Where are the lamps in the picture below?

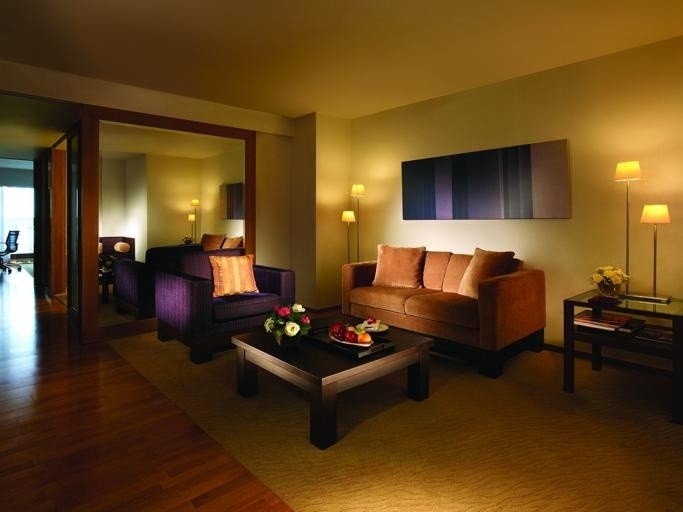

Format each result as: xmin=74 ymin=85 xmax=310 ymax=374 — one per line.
xmin=341 ymin=211 xmax=356 ymax=263
xmin=188 ymin=215 xmax=195 ymax=243
xmin=350 ymin=184 xmax=366 ymax=262
xmin=191 ymin=199 xmax=200 ymax=243
xmin=640 ymin=204 xmax=675 ymax=297
xmin=614 ymin=161 xmax=642 ymax=291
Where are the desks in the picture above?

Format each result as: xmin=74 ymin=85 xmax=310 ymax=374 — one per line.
xmin=98 ymin=276 xmax=112 ymax=305
xmin=563 ymin=288 xmax=683 ymax=426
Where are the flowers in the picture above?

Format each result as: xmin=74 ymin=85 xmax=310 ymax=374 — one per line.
xmin=264 ymin=303 xmax=312 ymax=338
xmin=591 ymin=265 xmax=629 ymax=287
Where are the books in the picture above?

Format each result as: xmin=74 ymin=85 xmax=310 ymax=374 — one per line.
xmin=572 ymin=309 xmax=645 ymax=341
xmin=634 ymin=324 xmax=672 ymax=344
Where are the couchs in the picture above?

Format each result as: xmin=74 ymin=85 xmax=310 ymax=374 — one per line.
xmin=341 ymin=260 xmax=546 ymax=378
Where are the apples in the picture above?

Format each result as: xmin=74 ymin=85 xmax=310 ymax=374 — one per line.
xmin=331 ymin=323 xmax=345 ymax=333
xmin=343 ymin=331 xmax=357 ymax=342
xmin=354 ymin=330 xmax=362 ymax=335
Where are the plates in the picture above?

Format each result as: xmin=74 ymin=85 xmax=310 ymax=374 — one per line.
xmin=356 ymin=323 xmax=389 ymax=332
xmin=329 ymin=328 xmax=374 ymax=347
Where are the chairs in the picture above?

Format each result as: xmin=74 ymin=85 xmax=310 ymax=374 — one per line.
xmin=113 ymin=246 xmax=200 ymax=319
xmin=0 ymin=231 xmax=21 ymax=274
xmin=154 ymin=266 xmax=295 ymax=364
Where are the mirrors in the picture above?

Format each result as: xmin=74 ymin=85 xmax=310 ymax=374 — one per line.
xmin=98 ymin=119 xmax=245 ymax=330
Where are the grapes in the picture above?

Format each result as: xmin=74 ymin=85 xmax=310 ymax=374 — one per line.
xmin=334 ymin=331 xmax=345 ymax=341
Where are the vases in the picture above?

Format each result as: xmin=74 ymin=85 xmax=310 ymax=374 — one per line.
xmin=599 ymin=282 xmax=620 ymax=296
xmin=273 ymin=335 xmax=294 ymax=347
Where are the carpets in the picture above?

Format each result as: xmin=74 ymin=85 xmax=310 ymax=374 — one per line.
xmin=108 ymin=311 xmax=683 ymax=512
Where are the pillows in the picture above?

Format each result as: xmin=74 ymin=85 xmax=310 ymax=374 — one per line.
xmin=201 ymin=234 xmax=226 ymax=251
xmin=222 ymin=237 xmax=243 ymax=248
xmin=367 ymin=244 xmax=515 ymax=301
xmin=208 ymin=254 xmax=260 ymax=298
xmin=177 ymin=249 xmax=246 ymax=303
